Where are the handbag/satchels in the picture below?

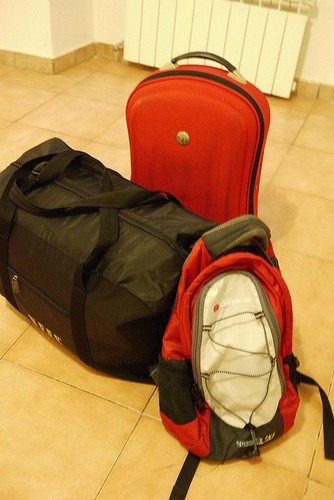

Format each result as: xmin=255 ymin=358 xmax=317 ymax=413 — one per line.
xmin=0 ymin=137 xmax=219 ymax=384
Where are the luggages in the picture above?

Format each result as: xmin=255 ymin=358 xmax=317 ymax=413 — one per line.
xmin=126 ymin=51 xmax=271 ymax=221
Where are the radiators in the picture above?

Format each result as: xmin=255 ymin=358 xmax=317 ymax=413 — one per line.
xmin=123 ymin=0 xmax=308 ymax=99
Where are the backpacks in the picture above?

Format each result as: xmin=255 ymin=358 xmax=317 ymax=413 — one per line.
xmin=158 ymin=215 xmax=300 ymax=461
